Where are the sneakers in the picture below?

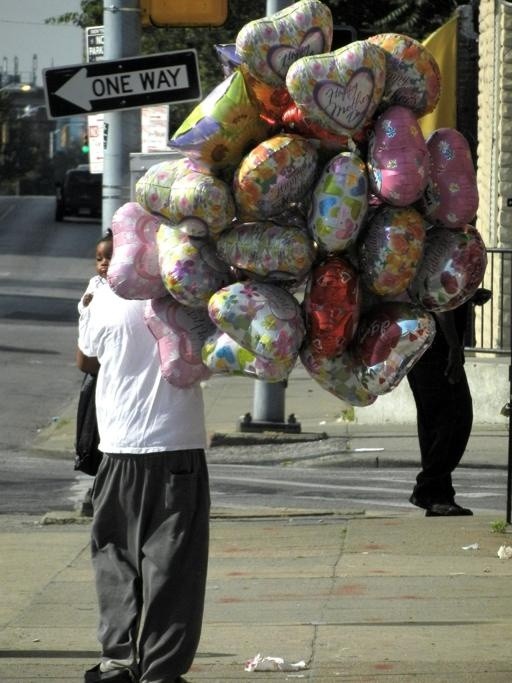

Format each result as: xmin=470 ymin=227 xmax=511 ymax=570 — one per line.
xmin=408 ymin=492 xmax=472 ymax=516
xmin=84 ymin=663 xmax=132 ymax=682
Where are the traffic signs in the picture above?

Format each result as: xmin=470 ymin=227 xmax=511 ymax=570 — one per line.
xmin=39 ymin=50 xmax=201 ymax=120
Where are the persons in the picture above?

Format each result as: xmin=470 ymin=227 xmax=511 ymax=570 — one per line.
xmin=77 ymin=228 xmax=114 ymax=312
xmin=75 ymin=281 xmax=213 ymax=683
xmin=407 ymin=288 xmax=490 ymax=518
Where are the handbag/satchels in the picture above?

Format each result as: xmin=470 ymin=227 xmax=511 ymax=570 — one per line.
xmin=74 ymin=370 xmax=104 ymax=476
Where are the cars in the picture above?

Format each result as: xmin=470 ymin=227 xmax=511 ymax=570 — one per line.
xmin=54 ymin=168 xmax=103 ymax=223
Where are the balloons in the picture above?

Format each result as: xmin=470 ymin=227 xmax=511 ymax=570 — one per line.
xmin=107 ymin=0 xmax=489 ymax=405
